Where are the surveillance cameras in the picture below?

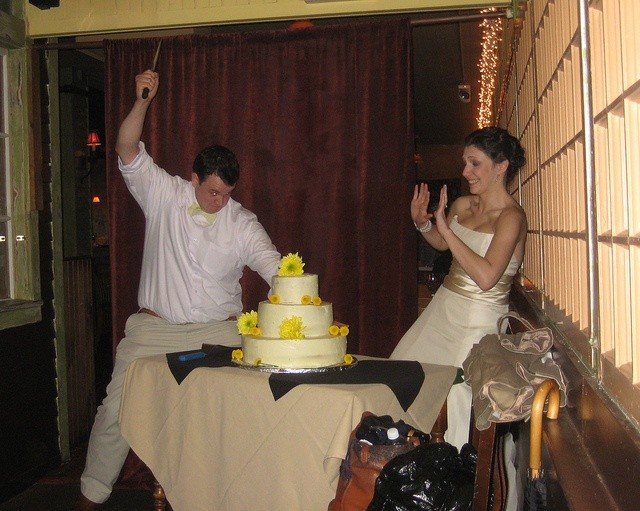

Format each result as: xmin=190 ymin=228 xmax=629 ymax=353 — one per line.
xmin=458 ymin=84 xmax=471 ymax=103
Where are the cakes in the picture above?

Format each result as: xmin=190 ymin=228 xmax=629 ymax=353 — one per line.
xmin=232 ymin=252 xmax=353 ymax=369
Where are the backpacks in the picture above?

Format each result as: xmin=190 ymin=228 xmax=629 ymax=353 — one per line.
xmin=367 ymin=444 xmax=479 ymax=510
xmin=327 ymin=408 xmax=429 ymax=509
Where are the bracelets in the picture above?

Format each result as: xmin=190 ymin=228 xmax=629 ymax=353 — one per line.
xmin=414 ymin=220 xmax=432 ymax=233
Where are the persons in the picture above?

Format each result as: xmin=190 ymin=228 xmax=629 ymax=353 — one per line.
xmin=384 ymin=126 xmax=529 ymax=454
xmin=77 ymin=69 xmax=283 ymax=511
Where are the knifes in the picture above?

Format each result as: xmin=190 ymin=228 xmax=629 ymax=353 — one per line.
xmin=141 ymin=41 xmax=163 ymax=100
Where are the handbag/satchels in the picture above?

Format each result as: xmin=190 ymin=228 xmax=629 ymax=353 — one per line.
xmin=461 ymin=312 xmax=568 ymax=431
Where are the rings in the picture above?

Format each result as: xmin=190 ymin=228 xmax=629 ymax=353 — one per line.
xmin=445 ymin=204 xmax=448 ymax=208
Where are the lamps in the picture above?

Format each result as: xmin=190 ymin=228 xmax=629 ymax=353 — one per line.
xmin=87 ymin=131 xmax=102 ymax=151
